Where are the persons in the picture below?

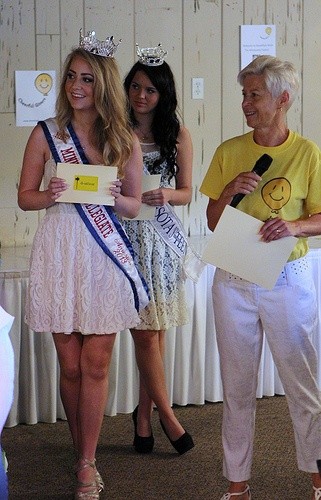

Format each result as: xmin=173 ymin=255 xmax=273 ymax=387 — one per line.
xmin=199 ymin=55 xmax=321 ymax=500
xmin=18 ymin=47 xmax=152 ymax=500
xmin=104 ymin=55 xmax=195 ymax=455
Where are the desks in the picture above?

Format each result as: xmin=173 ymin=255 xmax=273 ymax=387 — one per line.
xmin=0 ymin=234 xmax=321 ymax=428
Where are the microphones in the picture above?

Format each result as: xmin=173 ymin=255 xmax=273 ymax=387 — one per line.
xmin=230 ymin=153 xmax=273 ymax=208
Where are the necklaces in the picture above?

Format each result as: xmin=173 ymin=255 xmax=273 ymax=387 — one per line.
xmin=135 ymin=125 xmax=151 ymax=140
xmin=75 ymin=129 xmax=91 ymax=149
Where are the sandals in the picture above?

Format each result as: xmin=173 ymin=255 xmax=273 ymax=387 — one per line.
xmin=218 ymin=484 xmax=252 ymax=500
xmin=312 ymin=487 xmax=321 ymax=500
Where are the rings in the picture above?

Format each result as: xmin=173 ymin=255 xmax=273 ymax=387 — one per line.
xmin=276 ymin=228 xmax=280 ymax=233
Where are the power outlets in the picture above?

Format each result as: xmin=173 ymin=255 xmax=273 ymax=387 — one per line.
xmin=190 ymin=77 xmax=205 ymax=99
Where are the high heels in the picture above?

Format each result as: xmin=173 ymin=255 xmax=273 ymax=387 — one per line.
xmin=159 ymin=419 xmax=195 ymax=456
xmin=76 ymin=459 xmax=99 ymax=500
xmin=95 ymin=470 xmax=104 ymax=494
xmin=132 ymin=406 xmax=154 ymax=457
